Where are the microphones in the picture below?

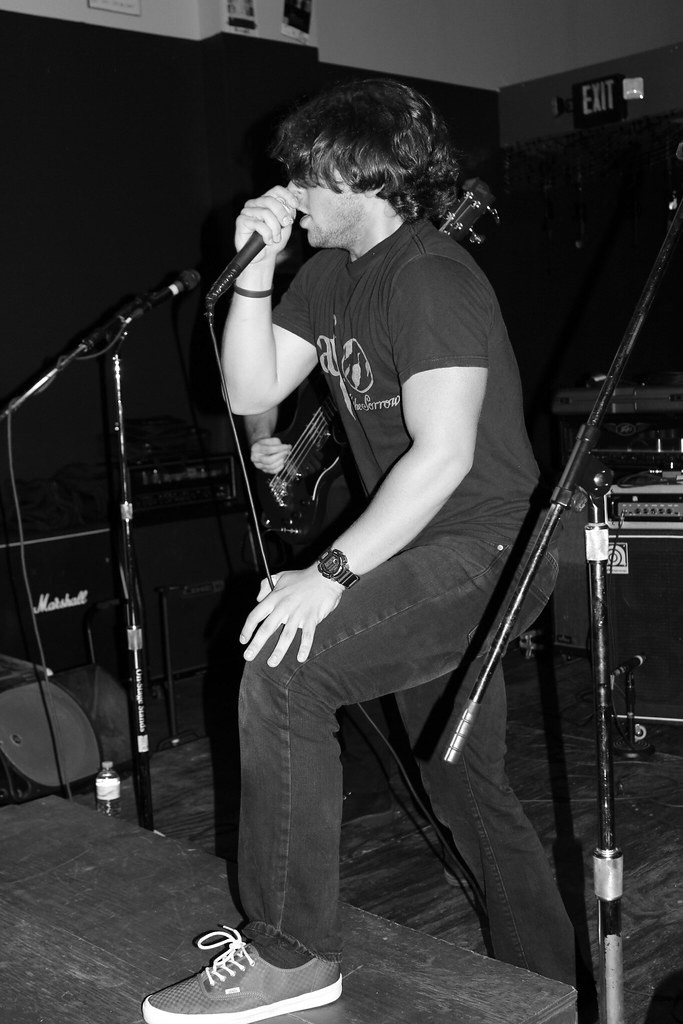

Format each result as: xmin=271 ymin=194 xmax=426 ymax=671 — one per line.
xmin=610 ymin=653 xmax=657 ymax=762
xmin=127 ymin=265 xmax=202 ymax=323
xmin=205 ymin=204 xmax=296 ymax=310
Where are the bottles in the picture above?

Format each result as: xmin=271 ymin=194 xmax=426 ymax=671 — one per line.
xmin=96 ymin=761 xmax=122 ymax=820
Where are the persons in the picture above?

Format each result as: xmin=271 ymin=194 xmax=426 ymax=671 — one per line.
xmin=142 ymin=82 xmax=603 ymax=1024
xmin=236 ymin=209 xmax=484 ymax=890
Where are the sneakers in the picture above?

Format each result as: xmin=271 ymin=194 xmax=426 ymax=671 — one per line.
xmin=140 ymin=926 xmax=343 ymax=1024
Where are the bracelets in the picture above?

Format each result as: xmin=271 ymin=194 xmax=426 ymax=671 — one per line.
xmin=234 ymin=285 xmax=273 ymax=299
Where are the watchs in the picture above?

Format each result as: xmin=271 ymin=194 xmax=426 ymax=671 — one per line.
xmin=320 ymin=548 xmax=360 ymax=590
